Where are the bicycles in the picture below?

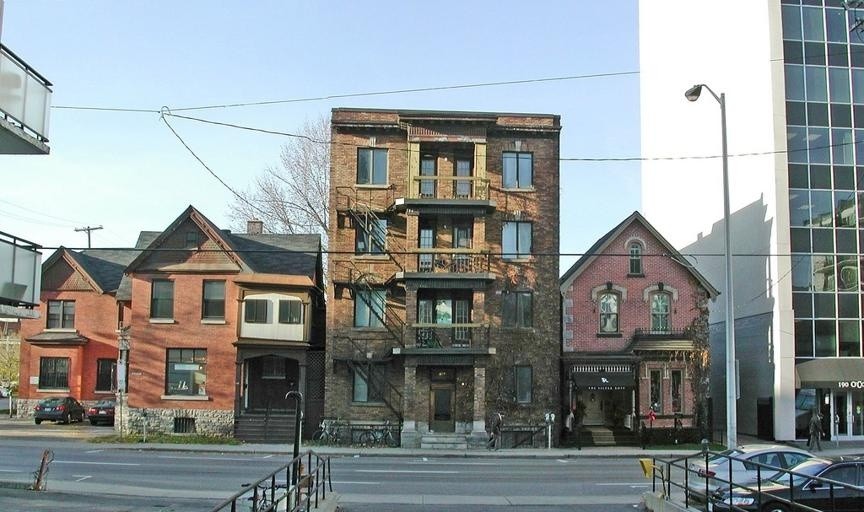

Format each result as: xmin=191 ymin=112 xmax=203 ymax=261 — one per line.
xmin=313 ymin=416 xmax=401 ymax=448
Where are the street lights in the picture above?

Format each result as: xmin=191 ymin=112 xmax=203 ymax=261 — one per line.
xmin=685 ymin=84 xmax=737 ymax=450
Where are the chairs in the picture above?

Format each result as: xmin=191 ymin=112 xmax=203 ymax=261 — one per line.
xmin=770 ymin=456 xmax=781 ymax=467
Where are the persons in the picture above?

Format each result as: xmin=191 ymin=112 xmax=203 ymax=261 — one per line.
xmin=809 ymin=413 xmax=826 ymax=453
xmin=485 ymin=411 xmax=505 ymax=452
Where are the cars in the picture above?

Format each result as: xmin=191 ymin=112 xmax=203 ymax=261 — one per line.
xmin=33 ymin=396 xmax=115 ymax=426
xmin=683 ymin=443 xmax=864 ymax=512
